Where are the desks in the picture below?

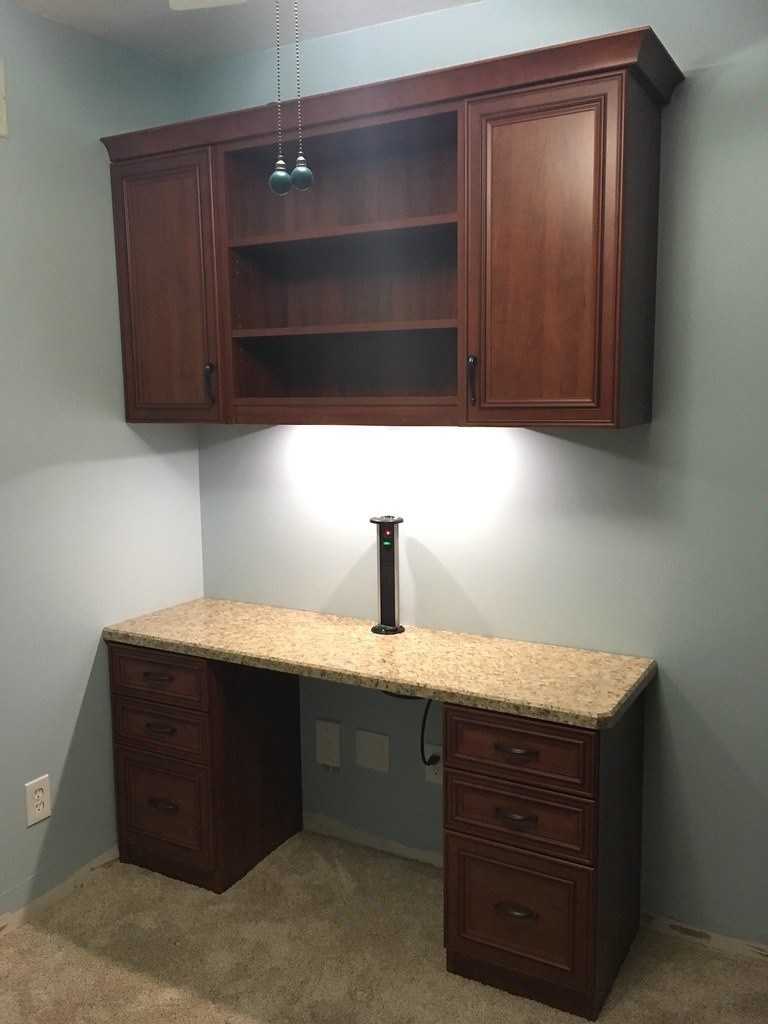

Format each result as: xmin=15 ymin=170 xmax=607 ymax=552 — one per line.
xmin=102 ymin=595 xmax=658 ymax=730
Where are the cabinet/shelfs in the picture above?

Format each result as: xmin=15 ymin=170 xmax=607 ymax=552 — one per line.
xmin=106 ymin=641 xmax=304 ymax=896
xmin=463 ymin=66 xmax=661 ymax=430
xmin=443 ymin=690 xmax=647 ymax=1022
xmin=227 ymin=211 xmax=464 ymax=407
xmin=110 ymin=145 xmax=227 ymax=425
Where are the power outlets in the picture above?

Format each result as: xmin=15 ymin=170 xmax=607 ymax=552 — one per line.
xmin=424 ymin=744 xmax=442 ymax=784
xmin=24 ymin=774 xmax=51 ymax=828
